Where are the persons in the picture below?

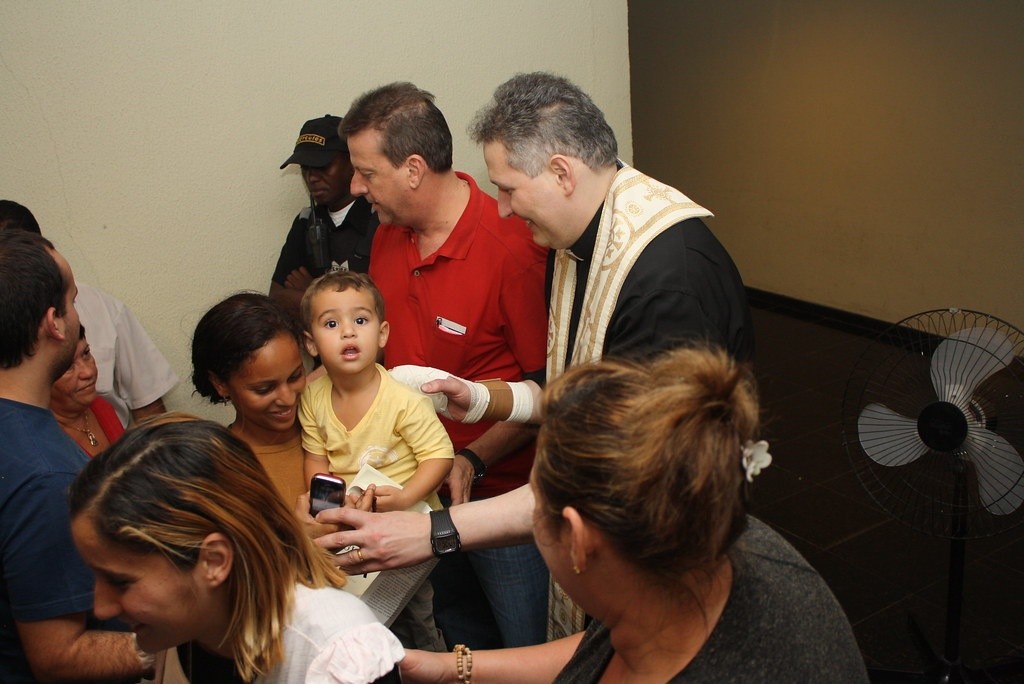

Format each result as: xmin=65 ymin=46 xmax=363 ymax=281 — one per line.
xmin=298 ymin=268 xmax=455 ymax=653
xmin=189 ymin=292 xmax=374 ymax=555
xmin=393 ymin=346 xmax=869 ymax=684
xmin=0 ymin=228 xmax=157 ymax=683
xmin=50 ymin=323 xmax=125 ymax=460
xmin=0 ymin=199 xmax=177 ymax=428
xmin=267 ymin=114 xmax=379 ymax=316
xmin=62 ymin=413 xmax=405 ymax=684
xmin=337 ymin=79 xmax=551 ymax=652
xmin=312 ymin=72 xmax=751 ymax=644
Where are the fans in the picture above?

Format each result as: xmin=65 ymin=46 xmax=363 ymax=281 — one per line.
xmin=837 ymin=306 xmax=1024 ymax=684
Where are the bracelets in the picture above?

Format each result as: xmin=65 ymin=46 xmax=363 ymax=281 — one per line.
xmin=452 ymin=644 xmax=473 ymax=684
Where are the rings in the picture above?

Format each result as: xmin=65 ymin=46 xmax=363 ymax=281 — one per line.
xmin=356 ymin=550 xmax=366 ymax=562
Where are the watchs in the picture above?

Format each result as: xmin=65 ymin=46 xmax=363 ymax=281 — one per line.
xmin=428 ymin=509 xmax=461 ymax=558
xmin=456 ymin=447 xmax=488 ymax=482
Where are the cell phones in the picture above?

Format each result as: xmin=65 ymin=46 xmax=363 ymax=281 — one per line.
xmin=308 ymin=473 xmax=346 ymax=518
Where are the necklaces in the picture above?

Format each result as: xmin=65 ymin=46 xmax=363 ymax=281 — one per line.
xmin=60 ymin=411 xmax=99 ymax=446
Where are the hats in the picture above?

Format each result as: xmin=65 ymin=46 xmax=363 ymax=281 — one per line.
xmin=279 ymin=114 xmax=347 ymax=169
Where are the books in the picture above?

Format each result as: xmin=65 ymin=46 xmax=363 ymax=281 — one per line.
xmin=335 ymin=462 xmax=440 ymax=630
